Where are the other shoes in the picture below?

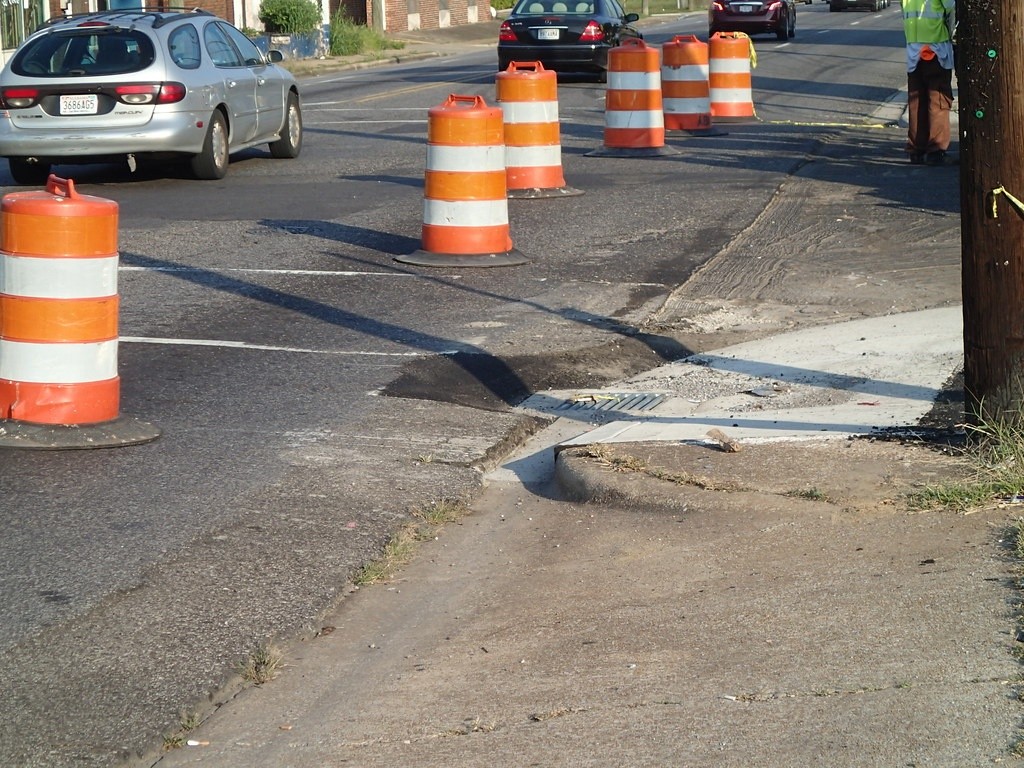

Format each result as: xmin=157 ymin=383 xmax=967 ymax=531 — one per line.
xmin=910 ymin=152 xmax=924 ymax=164
xmin=928 ymin=149 xmax=944 ymax=165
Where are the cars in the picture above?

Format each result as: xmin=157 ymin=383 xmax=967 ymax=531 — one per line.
xmin=827 ymin=0 xmax=891 ymax=12
xmin=708 ymin=0 xmax=797 ymax=39
xmin=498 ymin=0 xmax=643 ymax=82
xmin=0 ymin=7 xmax=304 ymax=181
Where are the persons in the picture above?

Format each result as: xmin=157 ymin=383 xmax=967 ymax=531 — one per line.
xmin=900 ymin=0 xmax=956 ymax=166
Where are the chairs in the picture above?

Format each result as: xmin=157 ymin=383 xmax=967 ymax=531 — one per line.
xmin=93 ymin=40 xmax=131 ymax=75
